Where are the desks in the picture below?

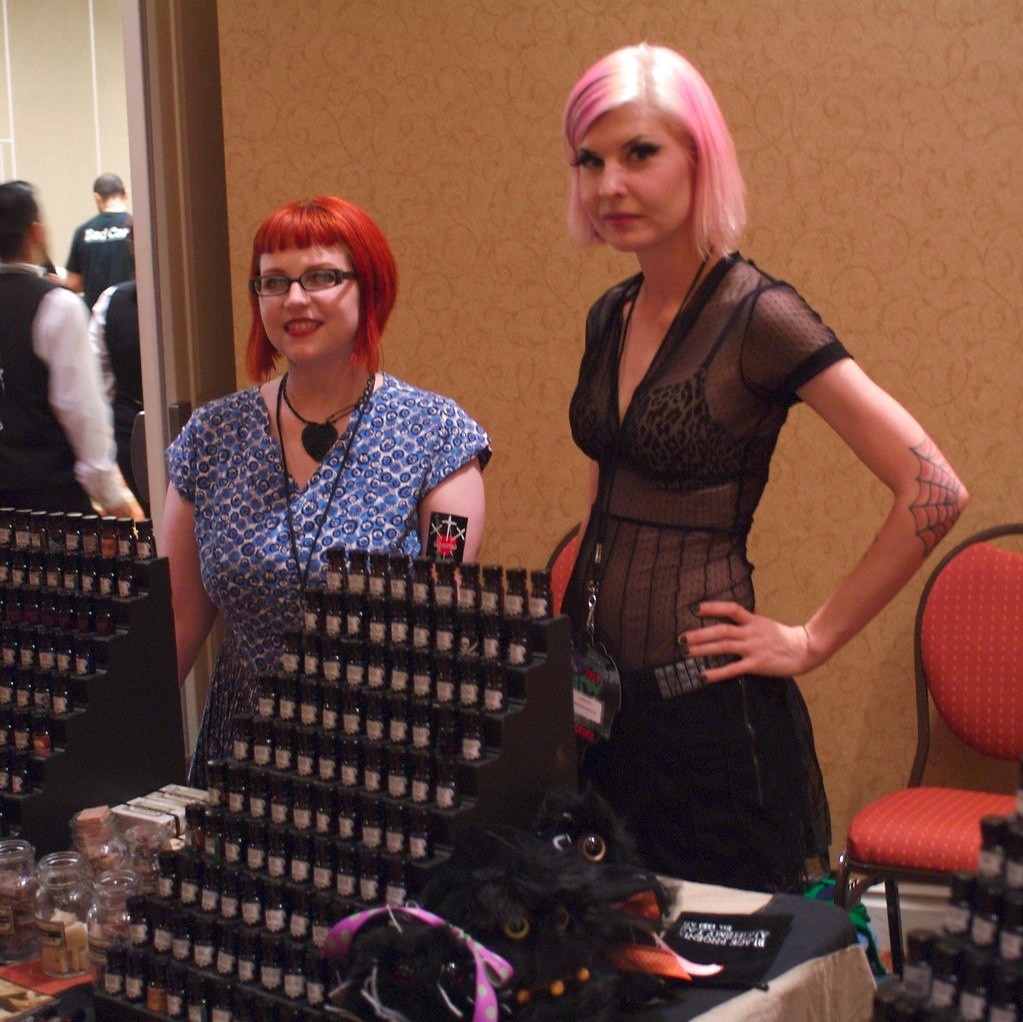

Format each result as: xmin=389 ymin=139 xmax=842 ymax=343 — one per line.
xmin=0 ymin=875 xmax=878 ymax=1022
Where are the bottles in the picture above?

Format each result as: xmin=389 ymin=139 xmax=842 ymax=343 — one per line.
xmin=0 ymin=814 xmax=169 ymax=980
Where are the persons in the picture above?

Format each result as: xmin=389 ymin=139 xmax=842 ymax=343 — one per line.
xmin=560 ymin=43 xmax=972 ymax=898
xmin=89 ymin=218 xmax=152 ymax=518
xmin=156 ymin=197 xmax=494 ymax=795
xmin=47 ymin=173 xmax=134 ymax=312
xmin=0 ymin=181 xmax=145 ymax=527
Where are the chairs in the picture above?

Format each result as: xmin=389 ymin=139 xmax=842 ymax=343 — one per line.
xmin=834 ymin=525 xmax=1023 ymax=980
xmin=546 ymin=523 xmax=583 ymax=618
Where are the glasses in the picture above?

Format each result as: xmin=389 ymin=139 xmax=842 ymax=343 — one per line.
xmin=249 ymin=266 xmax=359 ymax=297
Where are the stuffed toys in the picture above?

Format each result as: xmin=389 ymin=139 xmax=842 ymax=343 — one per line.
xmin=326 ymin=782 xmax=678 ymax=1022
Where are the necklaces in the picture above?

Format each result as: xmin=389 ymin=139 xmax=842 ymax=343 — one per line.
xmin=276 ymin=366 xmax=376 ymax=594
